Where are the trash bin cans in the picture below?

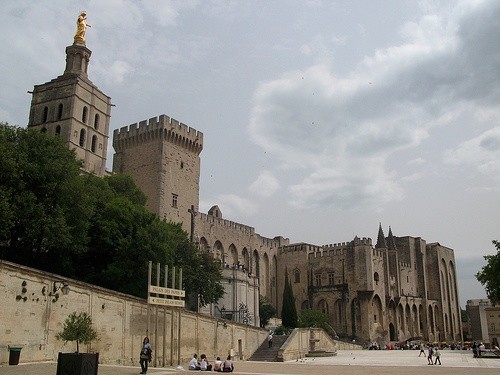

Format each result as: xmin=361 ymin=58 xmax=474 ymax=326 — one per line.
xmin=9 ymin=347 xmax=23 ymax=365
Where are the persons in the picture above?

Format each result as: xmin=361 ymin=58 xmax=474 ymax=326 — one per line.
xmin=418 ymin=342 xmax=426 ymax=357
xmin=200 ymin=354 xmax=212 ymax=371
xmin=268 ymin=333 xmax=273 ymax=348
xmin=189 ymin=353 xmax=201 ymax=371
xmin=248 ymin=272 xmax=253 ymax=279
xmin=362 ymin=343 xmax=398 ymax=350
xmin=283 ymin=332 xmax=285 ymax=335
xmin=139 ymin=337 xmax=151 ymax=375
xmin=223 ymin=261 xmax=244 ymax=270
xmin=399 ymin=342 xmax=450 ymax=350
xmin=428 ymin=345 xmax=434 ymax=365
xmin=450 ymin=342 xmax=485 ymax=356
xmin=223 ymin=355 xmax=234 ymax=372
xmin=75 ymin=10 xmax=91 ymax=41
xmin=493 ymin=344 xmax=500 ymax=351
xmin=214 ymin=357 xmax=222 ymax=371
xmin=434 ymin=347 xmax=441 ymax=365
xmin=243 ymin=267 xmax=247 ymax=271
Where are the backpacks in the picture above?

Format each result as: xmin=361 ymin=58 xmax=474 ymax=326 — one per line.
xmin=214 ymin=364 xmax=219 ymax=370
xmin=201 ymin=362 xmax=207 ymax=370
